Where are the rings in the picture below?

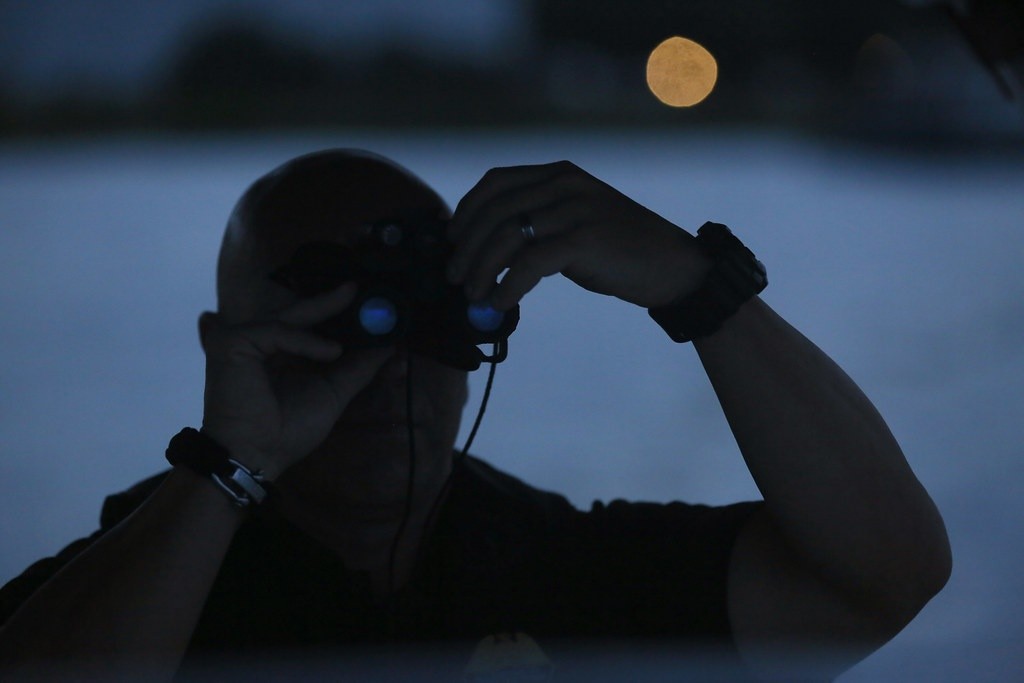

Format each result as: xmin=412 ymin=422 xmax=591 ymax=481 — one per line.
xmin=517 ymin=211 xmax=538 ymax=245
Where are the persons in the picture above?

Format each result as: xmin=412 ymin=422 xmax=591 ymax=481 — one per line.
xmin=1 ymin=149 xmax=949 ymax=681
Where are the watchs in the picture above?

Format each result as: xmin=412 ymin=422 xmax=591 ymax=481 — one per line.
xmin=648 ymin=221 xmax=768 ymax=343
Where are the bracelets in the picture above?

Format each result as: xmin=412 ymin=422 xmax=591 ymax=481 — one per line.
xmin=165 ymin=425 xmax=282 ymax=512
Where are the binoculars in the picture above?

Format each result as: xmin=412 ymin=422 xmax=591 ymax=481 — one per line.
xmin=281 ymin=206 xmax=521 ymax=366
